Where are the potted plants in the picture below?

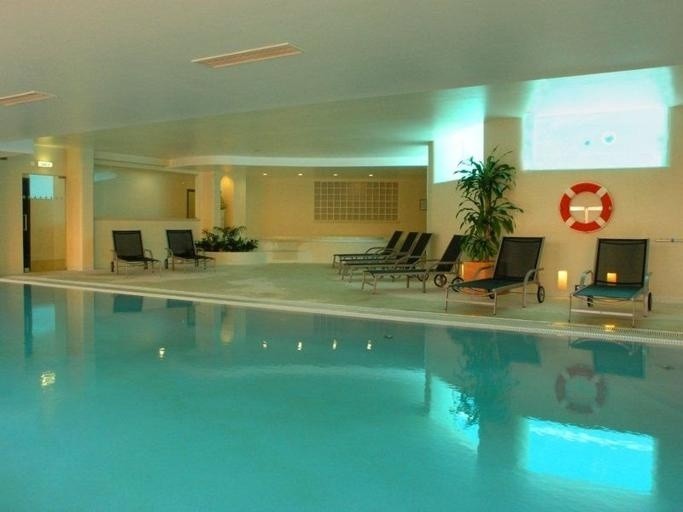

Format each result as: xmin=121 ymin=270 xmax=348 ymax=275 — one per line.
xmin=455 ymin=145 xmax=524 ymax=295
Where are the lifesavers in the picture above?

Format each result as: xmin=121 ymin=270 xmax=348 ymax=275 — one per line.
xmin=560 ymin=183 xmax=611 ymax=231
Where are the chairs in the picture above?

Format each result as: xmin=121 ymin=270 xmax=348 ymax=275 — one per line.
xmin=332 ymin=229 xmax=467 ymax=296
xmin=446 ymin=328 xmax=544 ymax=366
xmin=444 ymin=238 xmax=545 ymax=315
xmin=569 ymin=337 xmax=651 ymax=380
xmin=112 ymin=230 xmax=161 ymax=275
xmin=569 ymin=237 xmax=651 ymax=328
xmin=165 ymin=229 xmax=215 ymax=270
